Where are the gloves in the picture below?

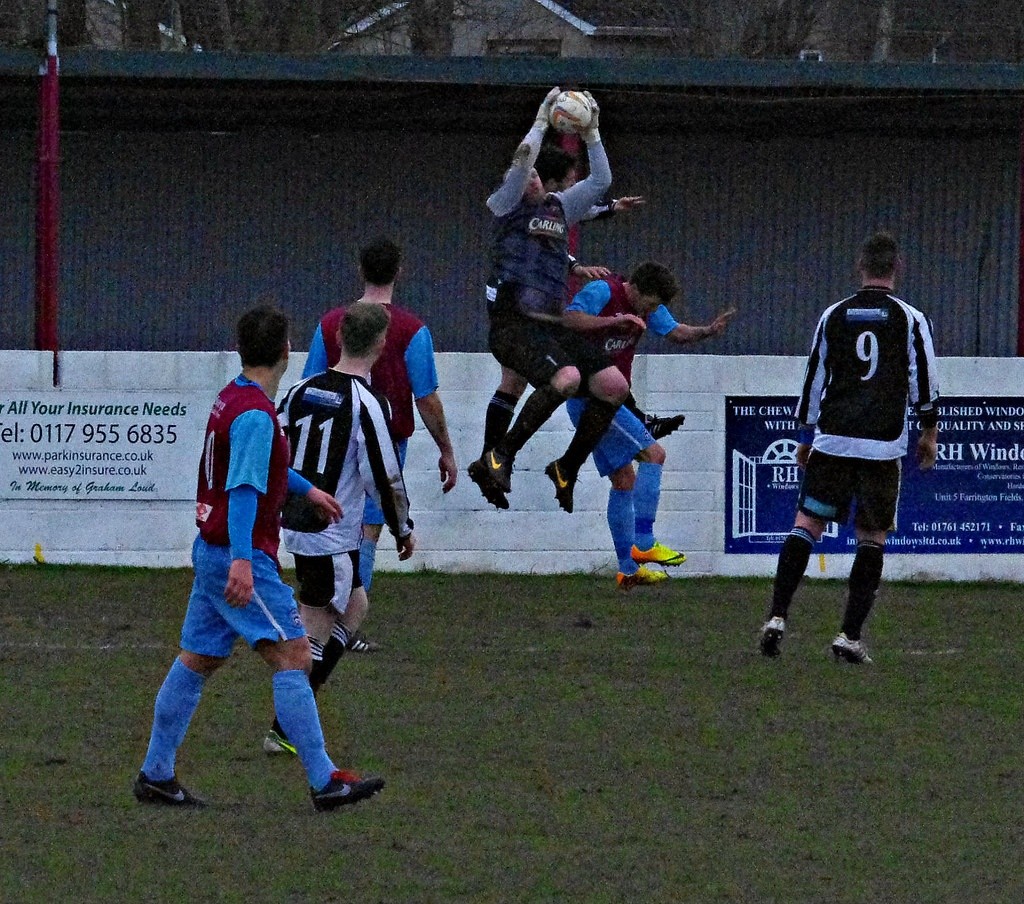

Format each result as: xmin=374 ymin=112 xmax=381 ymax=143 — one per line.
xmin=582 ymin=90 xmax=601 ymax=129
xmin=536 ymin=86 xmax=560 ymax=123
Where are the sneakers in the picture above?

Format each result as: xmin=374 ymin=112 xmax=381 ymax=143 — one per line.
xmin=484 ymin=447 xmax=512 ymax=492
xmin=263 ymin=729 xmax=298 ymax=755
xmin=347 ymin=635 xmax=379 ymax=652
xmin=467 ymin=460 xmax=509 ymax=509
xmin=630 ymin=539 xmax=686 ymax=567
xmin=134 ymin=771 xmax=209 ymax=808
xmin=310 ymin=770 xmax=386 ymax=812
xmin=831 ymin=633 xmax=873 ymax=664
xmin=545 ymin=460 xmax=583 ymax=513
xmin=643 ymin=414 xmax=685 ymax=440
xmin=760 ymin=616 xmax=786 ymax=658
xmin=616 ymin=563 xmax=670 ymax=591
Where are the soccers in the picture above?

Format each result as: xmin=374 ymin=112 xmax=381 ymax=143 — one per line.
xmin=550 ymin=91 xmax=592 ymax=133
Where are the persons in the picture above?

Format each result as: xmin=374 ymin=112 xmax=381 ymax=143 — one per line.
xmin=468 ymin=138 xmax=683 ymax=508
xmin=562 ymin=262 xmax=738 ymax=587
xmin=486 ymin=87 xmax=630 ymax=512
xmin=131 ymin=306 xmax=385 ymax=815
xmin=763 ymin=234 xmax=938 ymax=664
xmin=262 ymin=303 xmax=417 ymax=755
xmin=302 ymin=237 xmax=458 ymax=653
